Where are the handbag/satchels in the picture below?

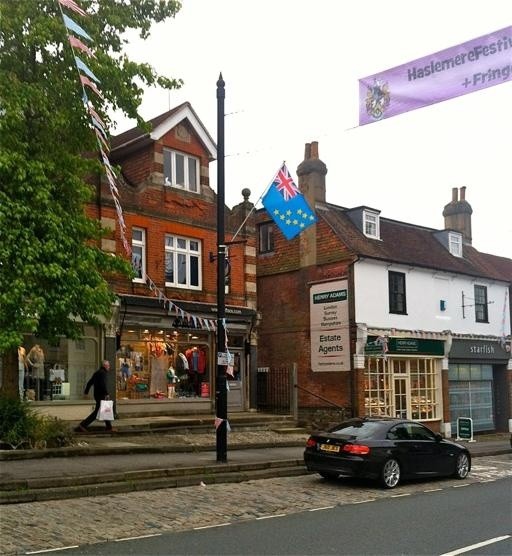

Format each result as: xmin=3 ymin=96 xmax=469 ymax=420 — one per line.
xmin=97 ymin=399 xmax=114 ymax=421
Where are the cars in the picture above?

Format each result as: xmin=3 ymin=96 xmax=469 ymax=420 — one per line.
xmin=303 ymin=416 xmax=472 ymax=490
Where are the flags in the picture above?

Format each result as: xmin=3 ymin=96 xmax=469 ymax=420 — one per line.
xmin=262 ymin=165 xmax=318 ymax=241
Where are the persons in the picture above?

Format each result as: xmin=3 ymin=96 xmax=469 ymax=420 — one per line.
xmin=176 ymin=353 xmax=190 ymax=380
xmin=18 ymin=344 xmax=29 ymax=402
xmin=185 ymin=346 xmax=206 ymax=374
xmin=165 ymin=365 xmax=181 ymax=400
xmin=27 ymin=344 xmax=46 ymax=381
xmin=78 ymin=359 xmax=118 ymax=434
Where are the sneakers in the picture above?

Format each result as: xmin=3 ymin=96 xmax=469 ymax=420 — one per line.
xmin=105 ymin=428 xmax=118 ymax=431
xmin=78 ymin=423 xmax=88 ymax=432
